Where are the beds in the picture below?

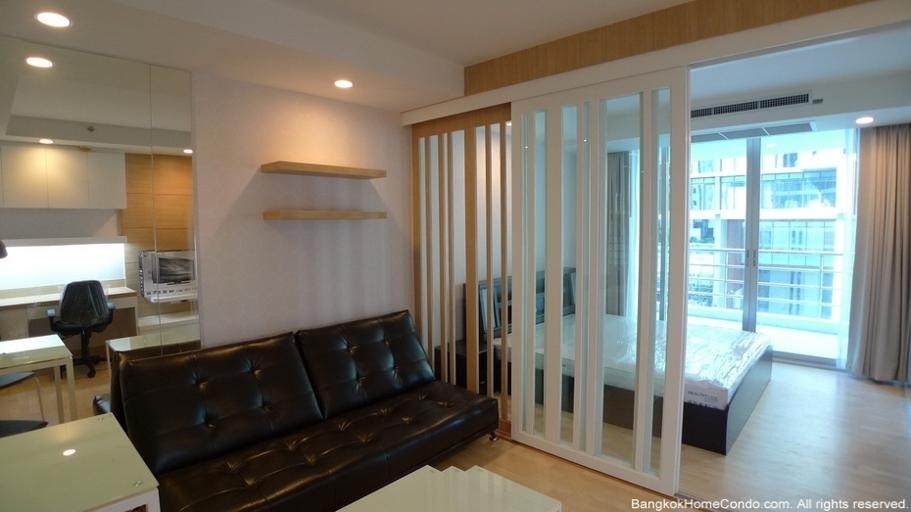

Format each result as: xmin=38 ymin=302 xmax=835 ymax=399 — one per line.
xmin=463 ymin=266 xmax=775 ymax=455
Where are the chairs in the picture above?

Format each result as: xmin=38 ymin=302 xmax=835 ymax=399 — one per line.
xmin=47 ymin=280 xmax=116 ymax=378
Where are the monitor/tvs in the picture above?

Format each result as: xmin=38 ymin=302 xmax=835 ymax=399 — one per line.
xmin=152 ymin=257 xmax=196 ymax=286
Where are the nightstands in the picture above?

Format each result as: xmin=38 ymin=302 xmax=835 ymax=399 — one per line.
xmin=434 ymin=339 xmax=498 ymax=396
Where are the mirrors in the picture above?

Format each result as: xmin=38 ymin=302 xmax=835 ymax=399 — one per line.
xmin=1 ymin=30 xmax=204 ymax=437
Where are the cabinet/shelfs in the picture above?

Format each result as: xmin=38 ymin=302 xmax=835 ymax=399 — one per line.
xmin=261 ymin=161 xmax=389 ymax=222
xmin=0 ymin=142 xmax=127 ymax=209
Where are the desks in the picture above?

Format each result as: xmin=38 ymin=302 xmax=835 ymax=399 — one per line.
xmin=3 ymin=412 xmax=163 ymax=512
xmin=0 ymin=324 xmax=201 ymax=424
xmin=1 ymin=286 xmax=140 ymax=345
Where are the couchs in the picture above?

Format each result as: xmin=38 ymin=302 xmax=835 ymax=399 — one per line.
xmin=121 ymin=307 xmax=501 ymax=512
